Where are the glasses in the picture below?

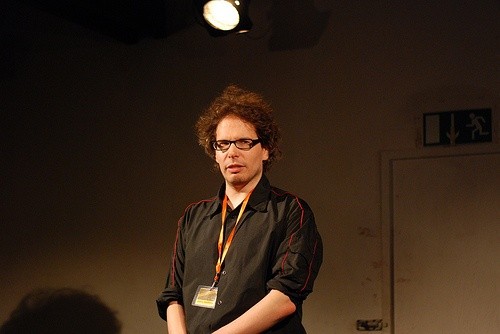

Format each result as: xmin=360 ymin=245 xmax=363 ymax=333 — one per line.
xmin=211 ymin=138 xmax=260 ymax=151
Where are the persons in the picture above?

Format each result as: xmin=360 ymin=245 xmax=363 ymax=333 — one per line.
xmin=156 ymin=86 xmax=324 ymax=334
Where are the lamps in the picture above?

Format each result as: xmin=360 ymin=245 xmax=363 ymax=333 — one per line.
xmin=202 ymin=0 xmax=252 ymax=36
